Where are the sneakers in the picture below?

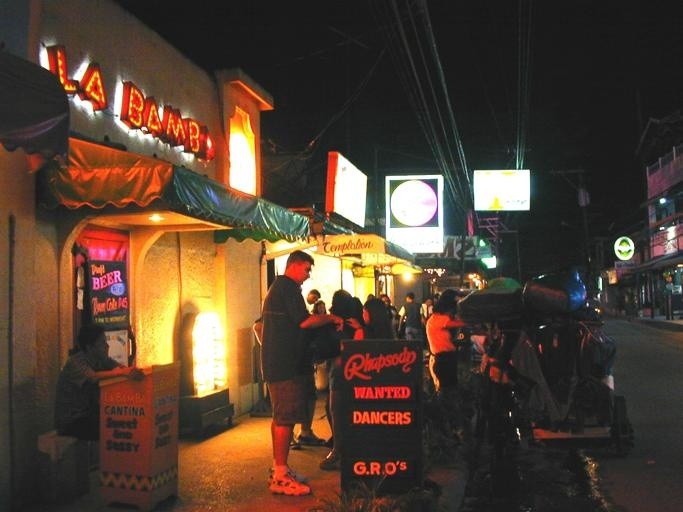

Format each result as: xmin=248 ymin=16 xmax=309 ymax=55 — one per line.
xmin=269 ymin=466 xmax=310 ymax=496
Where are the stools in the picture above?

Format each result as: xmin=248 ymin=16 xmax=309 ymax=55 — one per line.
xmin=38 ymin=431 xmax=97 ymax=511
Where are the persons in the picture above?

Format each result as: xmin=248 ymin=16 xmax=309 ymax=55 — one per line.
xmin=255 ymin=289 xmax=503 ymax=473
xmin=53 ymin=322 xmax=147 ymax=442
xmin=261 ymin=249 xmax=343 ymax=495
